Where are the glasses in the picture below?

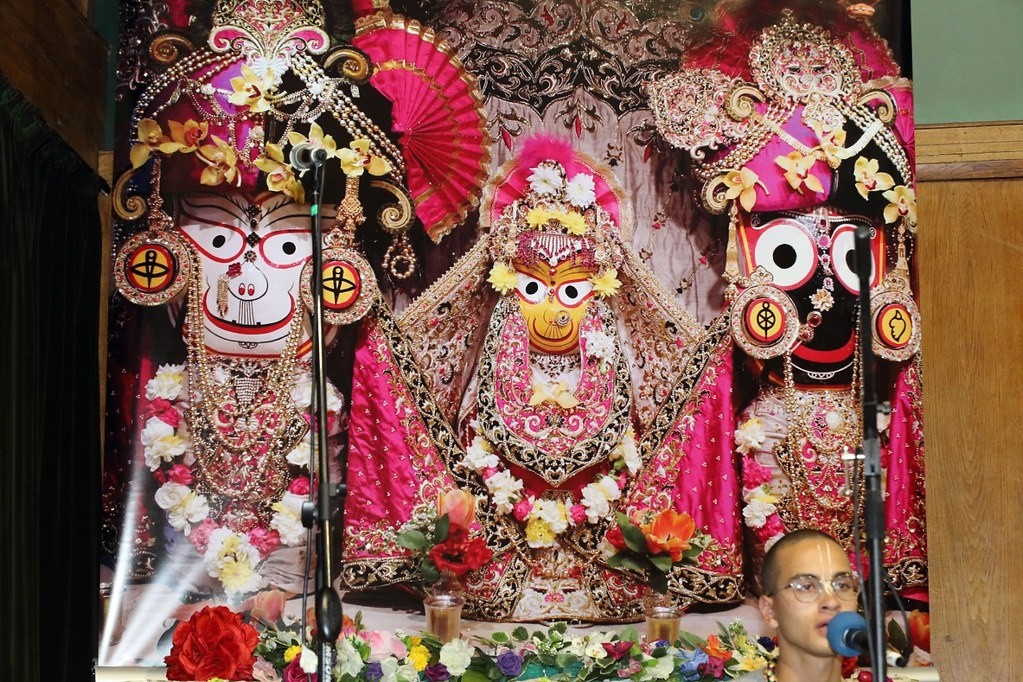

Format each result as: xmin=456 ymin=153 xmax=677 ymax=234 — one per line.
xmin=766 ymin=574 xmax=860 ymax=603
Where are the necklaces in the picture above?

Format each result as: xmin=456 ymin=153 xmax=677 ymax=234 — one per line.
xmin=766 ymin=652 xmax=872 ymax=682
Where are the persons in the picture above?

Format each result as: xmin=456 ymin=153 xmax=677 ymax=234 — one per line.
xmin=720 ymin=521 xmax=868 ymax=682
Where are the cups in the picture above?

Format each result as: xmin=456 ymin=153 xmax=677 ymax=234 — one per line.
xmin=644 ymin=607 xmax=685 ymax=648
xmin=422 ymin=595 xmax=464 ymax=643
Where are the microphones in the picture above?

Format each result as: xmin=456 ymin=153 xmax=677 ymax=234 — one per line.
xmin=826 ymin=610 xmax=907 ymax=667
xmin=289 ymin=142 xmax=327 ymax=172
